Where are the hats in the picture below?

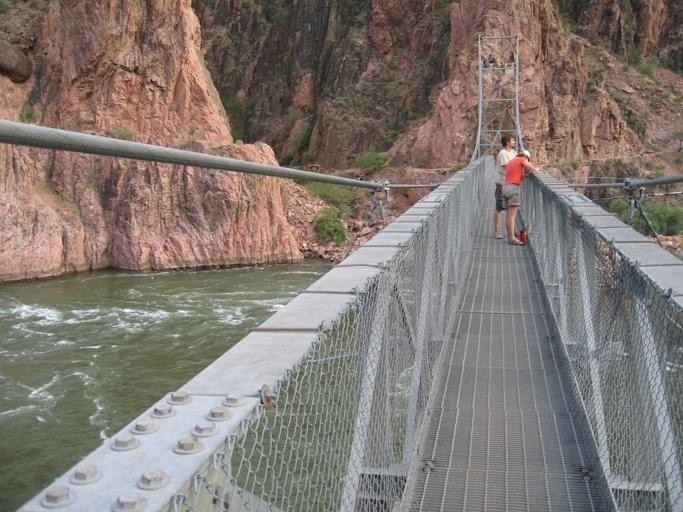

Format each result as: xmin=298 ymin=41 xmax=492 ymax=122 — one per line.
xmin=517 ymin=150 xmax=531 ymax=159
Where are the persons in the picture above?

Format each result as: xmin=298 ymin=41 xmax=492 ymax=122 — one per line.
xmin=493 ymin=135 xmax=517 ymax=240
xmin=501 ymin=150 xmax=541 ymax=246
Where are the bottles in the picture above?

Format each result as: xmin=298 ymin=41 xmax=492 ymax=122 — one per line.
xmin=520 ymin=230 xmax=527 ymax=243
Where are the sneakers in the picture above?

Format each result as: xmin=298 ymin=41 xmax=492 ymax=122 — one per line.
xmin=494 ymin=230 xmax=525 ymax=246
